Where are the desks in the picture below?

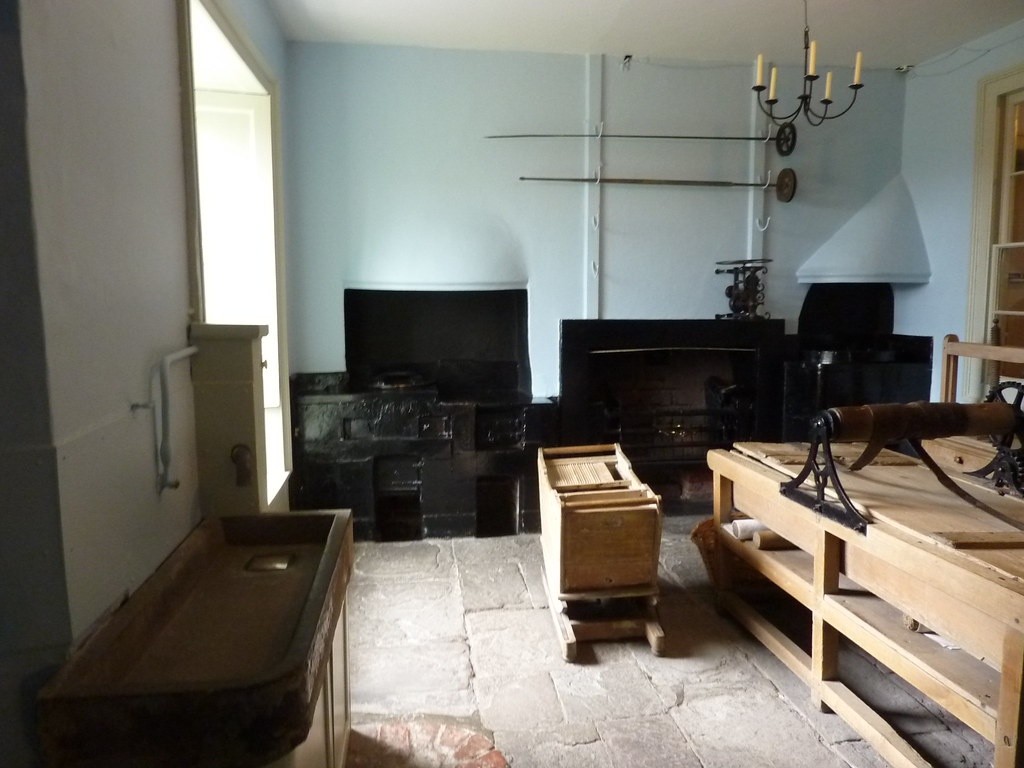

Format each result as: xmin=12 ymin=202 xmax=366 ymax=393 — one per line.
xmin=704 ymin=447 xmax=1024 ymax=767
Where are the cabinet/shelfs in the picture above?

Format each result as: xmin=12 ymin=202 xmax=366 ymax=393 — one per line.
xmin=266 ymin=586 xmax=356 ymax=768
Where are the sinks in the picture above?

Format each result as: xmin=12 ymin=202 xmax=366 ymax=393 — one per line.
xmin=26 ymin=509 xmax=356 ymax=768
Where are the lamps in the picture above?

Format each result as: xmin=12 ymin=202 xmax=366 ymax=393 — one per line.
xmin=750 ymin=0 xmax=864 ymax=126
